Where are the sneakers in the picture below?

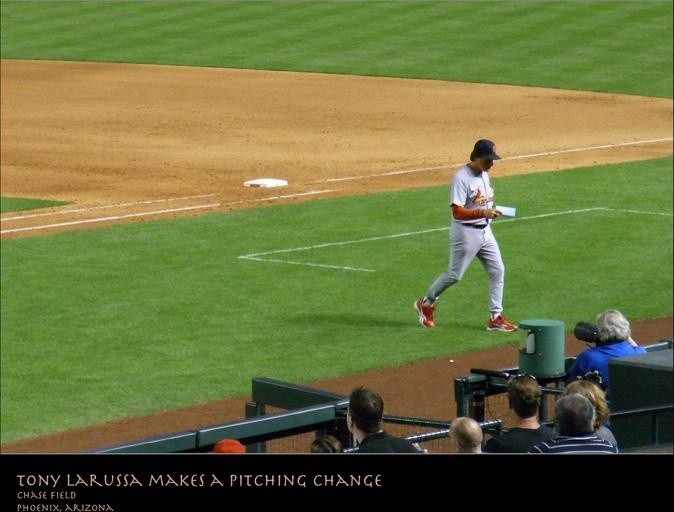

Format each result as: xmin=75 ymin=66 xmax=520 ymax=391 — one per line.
xmin=414 ymin=296 xmax=438 ymax=330
xmin=486 ymin=314 xmax=518 ymax=334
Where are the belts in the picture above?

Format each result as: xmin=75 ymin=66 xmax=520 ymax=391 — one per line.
xmin=463 ymin=223 xmax=489 ymax=230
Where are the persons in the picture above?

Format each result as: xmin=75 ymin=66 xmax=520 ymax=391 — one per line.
xmin=449 ymin=417 xmax=485 ymax=454
xmin=486 ymin=377 xmax=557 ymax=454
xmin=526 ymin=393 xmax=619 ymax=454
xmin=346 ymin=387 xmax=419 ymax=453
xmin=211 ymin=439 xmax=246 ymax=453
xmin=414 ymin=140 xmax=518 ymax=332
xmin=310 ymin=436 xmax=342 ymax=454
xmin=568 ymin=309 xmax=647 ymax=428
xmin=565 ymin=380 xmax=620 ymax=451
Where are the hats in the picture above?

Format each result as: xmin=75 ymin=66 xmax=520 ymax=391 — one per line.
xmin=470 ymin=138 xmax=502 ymax=162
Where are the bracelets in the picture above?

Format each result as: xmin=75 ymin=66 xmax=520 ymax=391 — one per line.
xmin=472 ymin=208 xmax=482 ymax=217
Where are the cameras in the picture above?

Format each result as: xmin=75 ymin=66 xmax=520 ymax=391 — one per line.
xmin=573 ymin=321 xmax=599 ymax=343
